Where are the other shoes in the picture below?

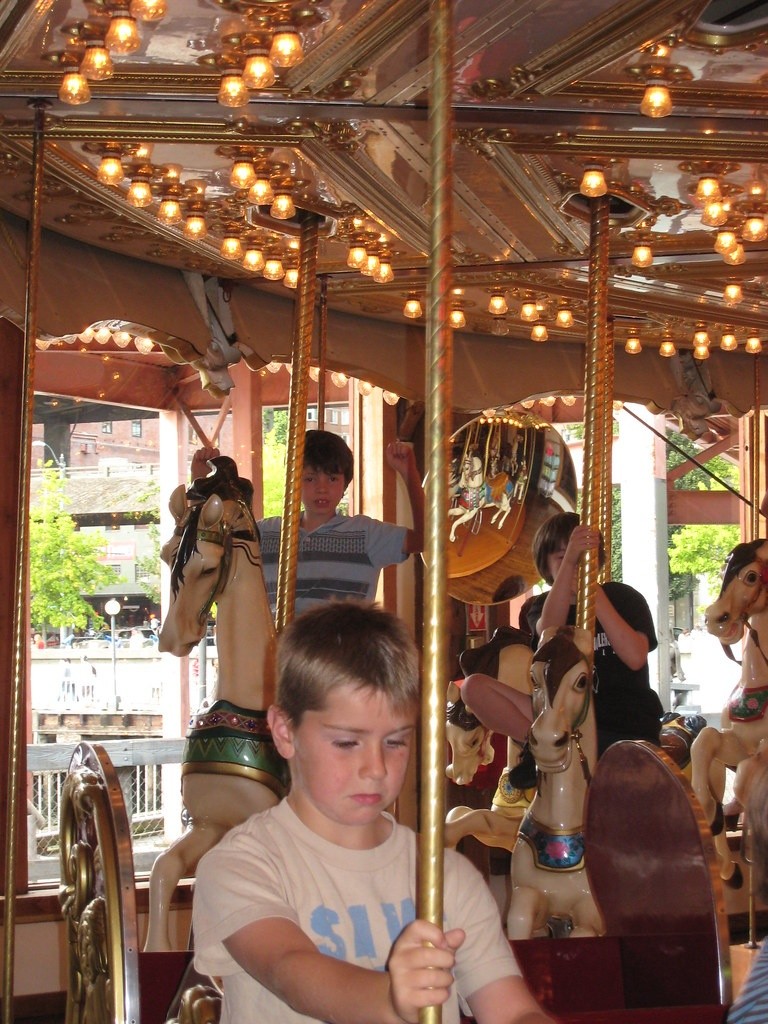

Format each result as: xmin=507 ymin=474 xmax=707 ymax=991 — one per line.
xmin=508 ymin=742 xmax=539 ymax=788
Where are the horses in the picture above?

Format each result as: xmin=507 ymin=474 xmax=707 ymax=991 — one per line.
xmin=144 ymin=455 xmax=768 ymax=954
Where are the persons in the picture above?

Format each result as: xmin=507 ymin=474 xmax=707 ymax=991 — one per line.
xmin=462 ymin=512 xmax=665 ymax=759
xmin=101 ymin=622 xmax=110 ymax=638
xmin=723 ymin=748 xmax=768 ymax=1024
xmin=191 ymin=601 xmax=554 ymax=1024
xmin=150 ymin=617 xmax=161 ymax=634
xmin=191 ymin=431 xmax=426 ymax=621
xmin=35 ymin=632 xmax=59 ymax=650
